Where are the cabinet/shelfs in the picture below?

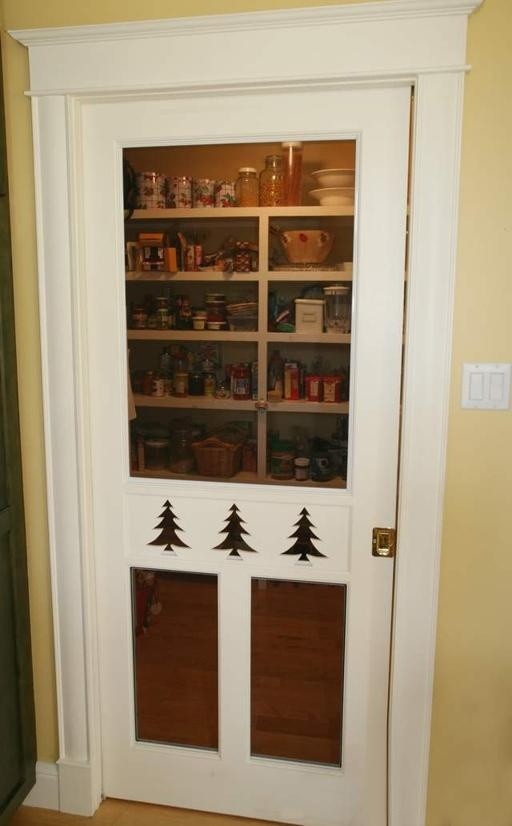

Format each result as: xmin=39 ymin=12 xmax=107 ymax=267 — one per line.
xmin=120 ymin=205 xmax=356 ymax=490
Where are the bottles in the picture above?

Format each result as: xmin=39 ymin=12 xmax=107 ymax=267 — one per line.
xmin=322 ymin=284 xmax=351 ymax=334
xmin=234 ymin=143 xmax=303 ymax=206
xmin=135 ymin=349 xmax=257 ymax=400
xmin=269 ymin=427 xmax=309 ymax=482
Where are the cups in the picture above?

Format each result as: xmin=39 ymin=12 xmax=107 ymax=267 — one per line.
xmin=131 ymin=296 xmax=190 ymax=329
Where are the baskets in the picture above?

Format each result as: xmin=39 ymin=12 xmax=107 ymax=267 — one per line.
xmin=191 ymin=438 xmax=243 ymax=477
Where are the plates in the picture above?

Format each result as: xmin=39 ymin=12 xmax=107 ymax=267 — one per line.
xmin=226 ymin=300 xmax=259 ymax=319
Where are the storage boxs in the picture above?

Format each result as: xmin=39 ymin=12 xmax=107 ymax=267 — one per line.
xmin=293 ymin=298 xmax=326 ymax=333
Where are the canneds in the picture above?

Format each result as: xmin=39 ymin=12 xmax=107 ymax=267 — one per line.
xmin=176 ymin=373 xmax=216 ymax=398
xmin=236 ymin=167 xmax=260 ymax=207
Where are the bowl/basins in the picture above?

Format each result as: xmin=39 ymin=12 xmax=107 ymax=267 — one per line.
xmin=204 ymin=292 xmax=226 ymax=331
xmin=308 ymin=167 xmax=355 ymax=187
xmin=308 ymin=186 xmax=355 ymax=206
xmin=279 ymin=229 xmax=336 ymax=265
